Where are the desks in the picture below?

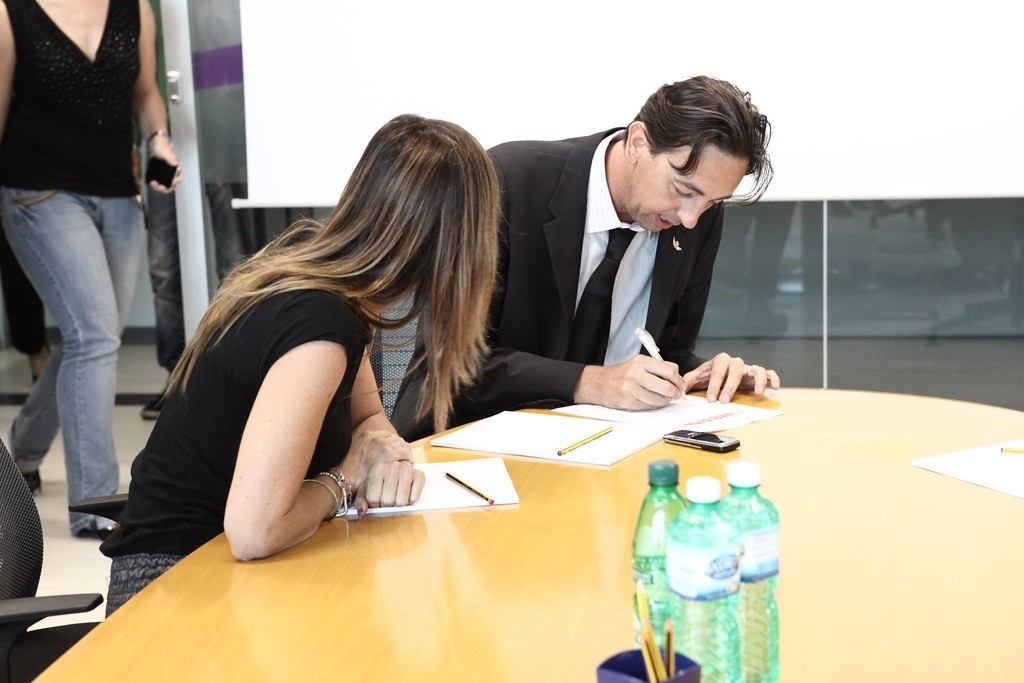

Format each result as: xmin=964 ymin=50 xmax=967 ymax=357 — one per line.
xmin=30 ymin=388 xmax=1024 ymax=683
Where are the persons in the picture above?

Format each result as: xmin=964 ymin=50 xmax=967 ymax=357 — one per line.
xmin=389 ymin=76 xmax=779 ymax=441
xmin=99 ymin=115 xmax=502 ymax=620
xmin=0 ymin=0 xmax=249 ymax=540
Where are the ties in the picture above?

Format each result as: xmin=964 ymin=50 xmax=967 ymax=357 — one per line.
xmin=564 ymin=228 xmax=638 ymax=366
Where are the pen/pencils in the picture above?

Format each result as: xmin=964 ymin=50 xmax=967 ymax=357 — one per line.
xmin=556 ymin=427 xmax=614 ymax=456
xmin=1001 ymin=448 xmax=1024 ymax=453
xmin=663 ymin=613 xmax=676 ymax=678
xmin=635 ymin=328 xmax=664 ymax=360
xmin=443 ymin=472 xmax=495 ymax=506
xmin=635 ymin=578 xmax=669 ymax=683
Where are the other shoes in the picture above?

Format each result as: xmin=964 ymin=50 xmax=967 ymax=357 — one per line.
xmin=140 ymin=389 xmax=168 ymax=420
xmin=73 ymin=515 xmax=119 ymax=542
xmin=15 ymin=458 xmax=41 ymax=494
xmin=32 ymin=341 xmax=52 ymax=383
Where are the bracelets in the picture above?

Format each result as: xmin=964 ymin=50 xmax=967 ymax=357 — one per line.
xmin=399 ymin=458 xmax=413 ymax=465
xmin=303 ymin=469 xmax=355 ymax=520
xmin=147 ymin=129 xmax=168 ymax=144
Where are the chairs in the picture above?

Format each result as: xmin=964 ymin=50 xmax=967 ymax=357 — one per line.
xmin=0 ymin=438 xmax=130 ymax=683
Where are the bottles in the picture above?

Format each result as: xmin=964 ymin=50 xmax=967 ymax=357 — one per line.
xmin=665 ymin=474 xmax=745 ymax=683
xmin=717 ymin=458 xmax=780 ymax=683
xmin=632 ymin=459 xmax=687 ymax=651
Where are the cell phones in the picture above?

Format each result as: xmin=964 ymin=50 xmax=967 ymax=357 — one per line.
xmin=145 ymin=157 xmax=181 ymax=190
xmin=663 ymin=429 xmax=740 ymax=453
